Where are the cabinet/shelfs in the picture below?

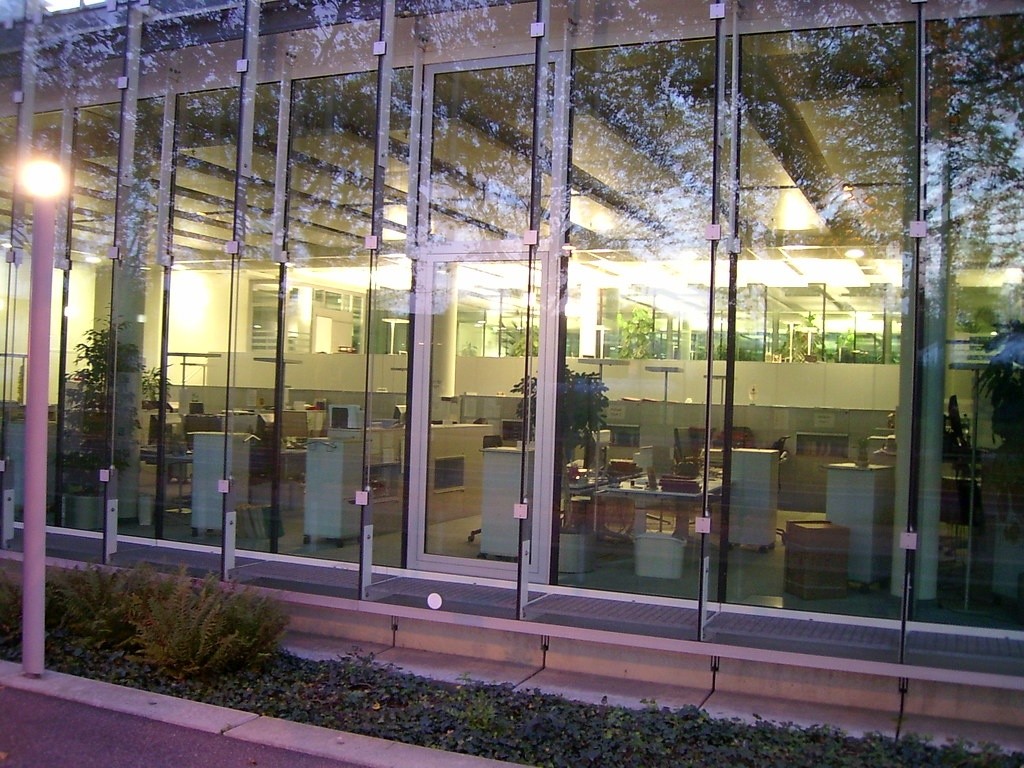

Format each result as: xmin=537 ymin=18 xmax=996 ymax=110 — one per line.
xmin=191 ymin=431 xmax=251 ymax=537
xmin=326 ymin=423 xmax=495 ymax=537
xmin=303 ymin=436 xmax=372 ymax=546
xmin=6 ymin=416 xmax=57 ymax=520
xmin=477 ymin=445 xmax=534 ymax=563
xmin=728 ymin=447 xmax=779 ymax=552
xmin=139 ymin=408 xmax=171 ymax=449
xmin=282 ymin=409 xmax=327 ymax=433
xmin=826 ymin=461 xmax=897 ymax=596
xmin=991 ymin=486 xmax=1024 ymax=617
xmin=221 ymin=409 xmax=257 ymax=433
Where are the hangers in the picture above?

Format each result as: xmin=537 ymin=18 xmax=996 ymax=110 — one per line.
xmin=244 ymin=428 xmax=262 ymax=443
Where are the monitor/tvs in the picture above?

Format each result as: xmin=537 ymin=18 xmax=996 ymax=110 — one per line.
xmin=148 ymin=413 xmax=183 ymax=446
xmin=281 ymin=410 xmax=308 ymax=441
xmin=675 ymin=427 xmax=697 ymax=460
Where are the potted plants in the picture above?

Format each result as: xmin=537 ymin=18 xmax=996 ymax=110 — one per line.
xmin=263 ymin=402 xmax=273 ymax=414
xmin=510 ymin=364 xmax=609 ymax=573
xmin=63 ymin=308 xmax=146 ymax=530
xmin=853 ymin=437 xmax=872 ymax=466
xmin=142 ymin=362 xmax=174 ymax=410
xmin=190 ymin=392 xmax=204 ymax=416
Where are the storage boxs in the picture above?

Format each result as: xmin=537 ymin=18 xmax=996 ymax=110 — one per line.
xmin=784 ymin=520 xmax=849 ymax=600
xmin=633 ymin=530 xmax=687 ymax=580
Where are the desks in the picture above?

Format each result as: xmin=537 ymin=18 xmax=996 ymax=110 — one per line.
xmin=559 ymin=458 xmax=723 ymax=565
xmin=183 ymin=414 xmax=222 ymax=449
xmin=260 ymin=414 xmax=274 ymax=432
xmin=140 ymin=445 xmax=193 ymax=527
xmin=701 ymin=445 xmax=724 ymax=468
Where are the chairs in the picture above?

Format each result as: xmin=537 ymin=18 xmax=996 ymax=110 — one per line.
xmin=466 ymin=435 xmax=504 ymax=543
xmin=938 ymin=476 xmax=988 ymax=577
xmin=282 ymin=410 xmax=309 ymax=482
xmin=676 ymin=426 xmax=701 ymax=460
xmin=770 ymin=436 xmax=791 ymax=467
